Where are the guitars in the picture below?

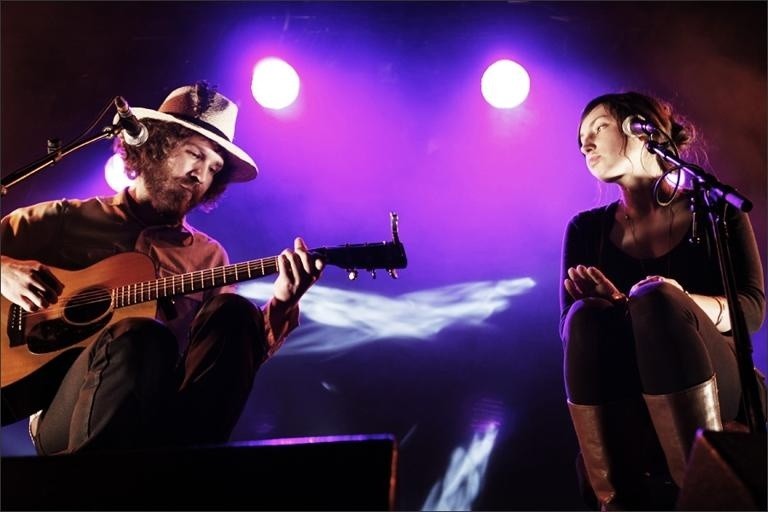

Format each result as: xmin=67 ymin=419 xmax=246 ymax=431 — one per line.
xmin=0 ymin=212 xmax=407 ymax=389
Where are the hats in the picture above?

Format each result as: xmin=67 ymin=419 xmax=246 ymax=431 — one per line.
xmin=115 ymin=83 xmax=258 ymax=183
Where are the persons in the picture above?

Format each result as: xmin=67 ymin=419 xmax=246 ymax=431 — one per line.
xmin=560 ymin=92 xmax=766 ymax=511
xmin=1 ymin=82 xmax=325 ymax=457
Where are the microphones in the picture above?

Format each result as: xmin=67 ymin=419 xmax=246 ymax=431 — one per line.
xmin=622 ymin=112 xmax=659 ymax=137
xmin=113 ymin=95 xmax=150 ymax=146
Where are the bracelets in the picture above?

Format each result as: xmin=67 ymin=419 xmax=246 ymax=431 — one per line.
xmin=714 ymin=296 xmax=725 ymax=327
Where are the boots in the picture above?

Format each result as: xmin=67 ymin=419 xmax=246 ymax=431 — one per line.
xmin=565 ymin=398 xmax=654 ymax=512
xmin=642 ymin=372 xmax=722 ymax=489
